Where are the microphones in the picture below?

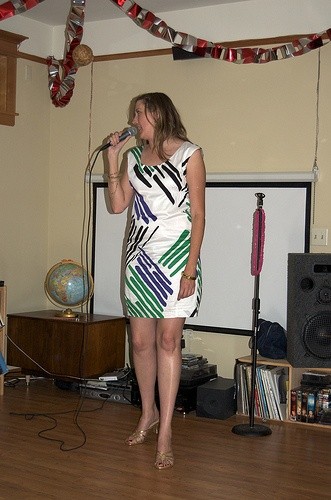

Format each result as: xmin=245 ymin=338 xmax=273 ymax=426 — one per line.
xmin=100 ymin=127 xmax=137 ymax=151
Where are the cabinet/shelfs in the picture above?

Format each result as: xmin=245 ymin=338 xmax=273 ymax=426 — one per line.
xmin=7 ymin=310 xmax=126 ymax=385
xmin=236 ymin=354 xmax=331 ymax=429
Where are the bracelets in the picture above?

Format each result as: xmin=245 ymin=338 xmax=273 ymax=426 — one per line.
xmin=181 ymin=270 xmax=198 ymax=282
xmin=107 ymin=171 xmax=119 ymax=199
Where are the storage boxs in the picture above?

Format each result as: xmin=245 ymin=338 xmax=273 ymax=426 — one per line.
xmin=196 ymin=379 xmax=236 ymax=419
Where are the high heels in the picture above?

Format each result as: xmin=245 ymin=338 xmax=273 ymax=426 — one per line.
xmin=126 ymin=420 xmax=160 ymax=445
xmin=155 ymin=450 xmax=175 ymax=469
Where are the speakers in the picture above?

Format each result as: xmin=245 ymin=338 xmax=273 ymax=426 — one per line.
xmin=287 ymin=253 xmax=331 ymax=369
xmin=196 ymin=377 xmax=237 ymax=419
xmin=172 ymin=46 xmax=201 ymax=60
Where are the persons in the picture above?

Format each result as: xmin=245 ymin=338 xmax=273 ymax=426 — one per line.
xmin=107 ymin=92 xmax=207 ymax=470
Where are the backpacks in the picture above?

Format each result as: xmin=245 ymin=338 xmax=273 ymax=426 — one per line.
xmin=256 ymin=319 xmax=287 ymax=359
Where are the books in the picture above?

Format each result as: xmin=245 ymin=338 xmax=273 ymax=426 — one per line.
xmin=238 ymin=364 xmax=289 ymax=420
